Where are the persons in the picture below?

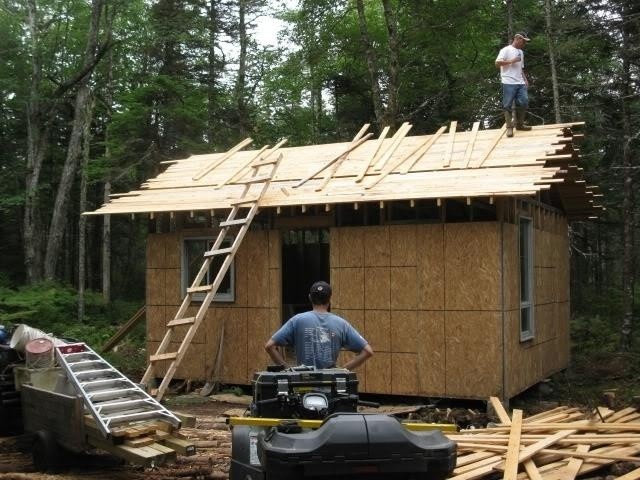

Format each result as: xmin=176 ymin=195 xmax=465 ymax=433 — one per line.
xmin=264 ymin=280 xmax=374 ymax=371
xmin=494 ymin=31 xmax=532 ymax=137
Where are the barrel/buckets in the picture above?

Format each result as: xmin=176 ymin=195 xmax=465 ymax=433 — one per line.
xmin=10 ymin=324 xmax=70 ymax=353
xmin=25 ymin=338 xmax=54 ymax=368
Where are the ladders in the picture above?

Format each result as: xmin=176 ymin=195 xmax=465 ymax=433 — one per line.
xmin=53 ymin=342 xmax=183 ymax=439
xmin=139 ymin=153 xmax=284 ymax=402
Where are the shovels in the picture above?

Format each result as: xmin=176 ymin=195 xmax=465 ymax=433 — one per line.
xmin=198 ymin=320 xmax=225 ymax=396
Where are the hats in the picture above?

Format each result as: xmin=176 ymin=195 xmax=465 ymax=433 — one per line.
xmin=513 ymin=31 xmax=532 ymax=42
xmin=312 ymin=281 xmax=331 ymax=294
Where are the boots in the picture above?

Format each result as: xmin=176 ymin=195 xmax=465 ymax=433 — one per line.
xmin=516 ymin=108 xmax=531 ymax=131
xmin=504 ymin=111 xmax=513 ymax=138
